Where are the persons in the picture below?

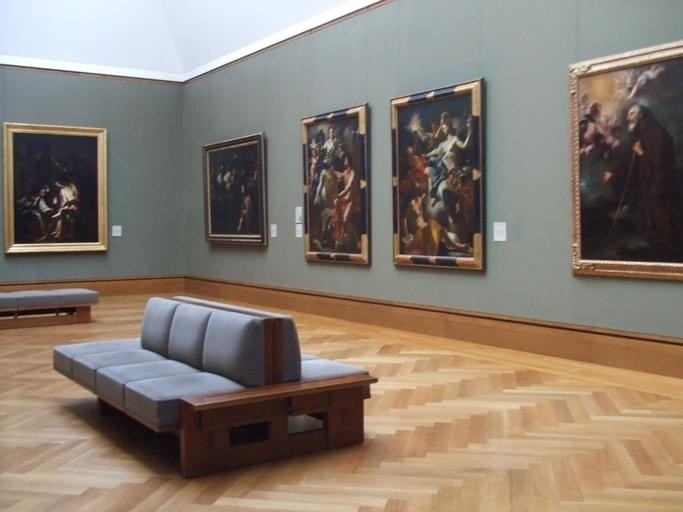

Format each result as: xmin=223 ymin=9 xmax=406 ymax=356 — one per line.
xmin=23 ymin=182 xmax=55 ymax=214
xmin=238 ymin=184 xmax=256 ymax=234
xmin=400 ymin=110 xmax=476 ymax=255
xmin=310 ymin=126 xmax=362 ymax=252
xmin=578 ymin=92 xmax=683 ymax=262
xmin=38 ymin=179 xmax=80 ymax=241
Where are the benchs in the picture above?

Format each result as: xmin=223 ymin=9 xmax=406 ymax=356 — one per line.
xmin=52 ymin=297 xmax=270 ymax=471
xmin=172 ymin=296 xmax=378 ymax=458
xmin=0 ymin=287 xmax=100 ymax=330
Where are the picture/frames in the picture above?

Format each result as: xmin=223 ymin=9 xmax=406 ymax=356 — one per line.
xmin=2 ymin=122 xmax=108 ymax=254
xmin=202 ymin=132 xmax=268 ymax=248
xmin=567 ymin=40 xmax=683 ymax=282
xmin=390 ymin=78 xmax=488 ymax=272
xmin=301 ymin=103 xmax=371 ymax=267
